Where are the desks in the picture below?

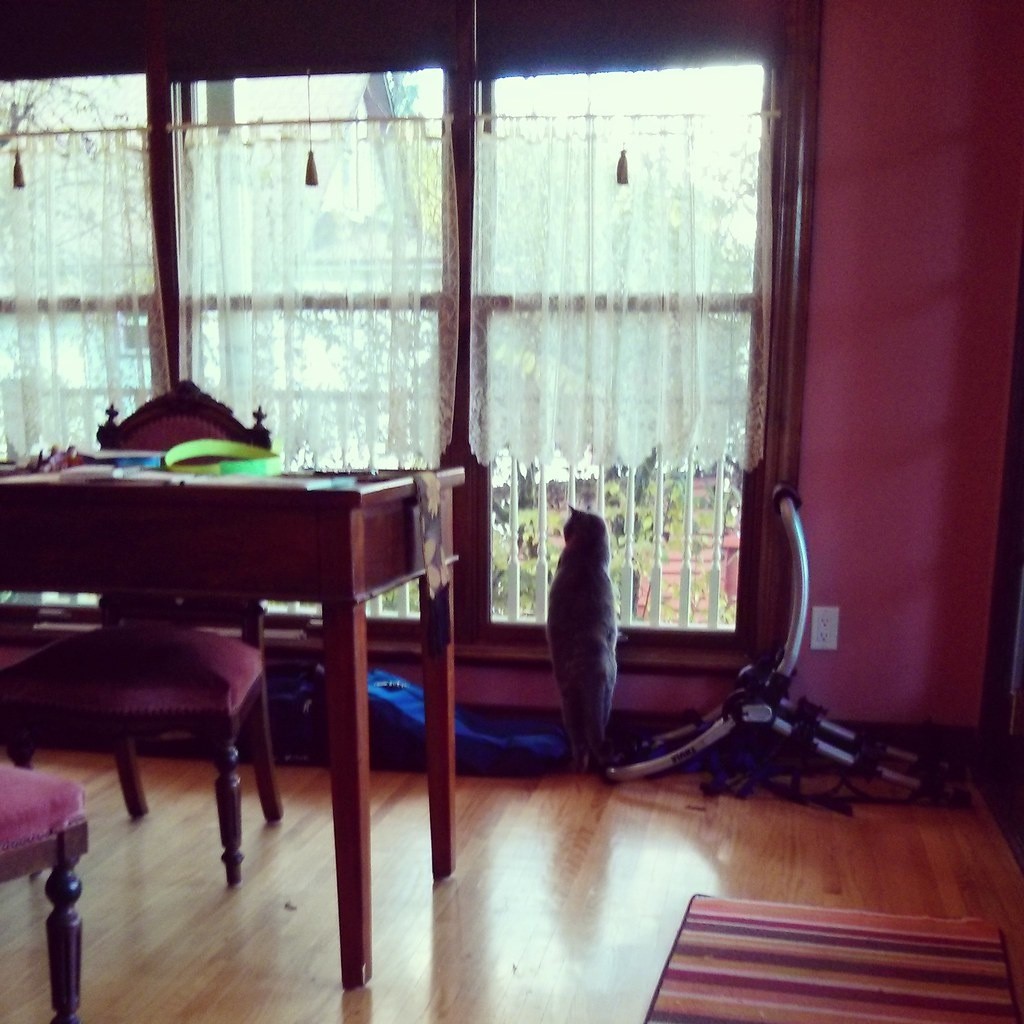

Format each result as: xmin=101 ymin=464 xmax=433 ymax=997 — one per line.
xmin=0 ymin=465 xmax=470 ymax=987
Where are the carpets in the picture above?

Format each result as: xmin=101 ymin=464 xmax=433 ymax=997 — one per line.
xmin=643 ymin=893 xmax=1021 ymax=1024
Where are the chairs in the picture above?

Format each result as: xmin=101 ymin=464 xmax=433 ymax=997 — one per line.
xmin=0 ymin=377 xmax=285 ymax=887
xmin=0 ymin=764 xmax=90 ymax=1024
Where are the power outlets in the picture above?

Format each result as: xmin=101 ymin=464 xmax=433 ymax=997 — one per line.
xmin=810 ymin=605 xmax=840 ymax=650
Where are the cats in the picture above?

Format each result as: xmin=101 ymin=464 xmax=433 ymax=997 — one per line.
xmin=546 ymin=506 xmax=627 ymax=772
